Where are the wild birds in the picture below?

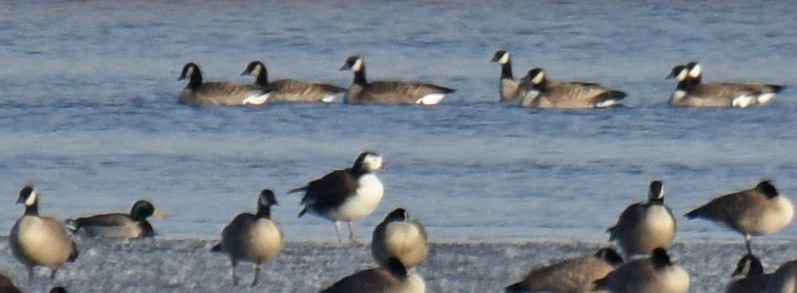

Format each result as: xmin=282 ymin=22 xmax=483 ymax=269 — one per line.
xmin=210 ymin=189 xmax=285 ymax=289
xmin=239 ymin=60 xmax=349 ymax=103
xmin=501 ymin=246 xmax=797 ymax=293
xmin=65 ymin=200 xmax=167 ymax=240
xmin=340 ymin=56 xmax=457 ymax=104
xmin=685 ymin=179 xmax=794 ymax=254
xmin=664 ymin=62 xmax=792 ymax=107
xmin=176 ymin=62 xmax=275 ymax=105
xmin=319 ymin=208 xmax=428 ymax=293
xmin=607 ymin=180 xmax=677 ymax=254
xmin=288 ymin=149 xmax=389 ymax=248
xmin=9 ymin=186 xmax=79 ymax=282
xmin=490 ymin=50 xmax=628 ymax=111
